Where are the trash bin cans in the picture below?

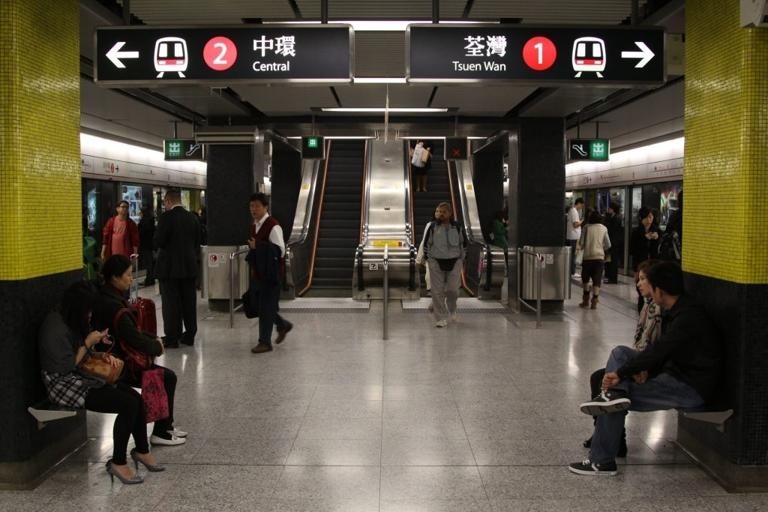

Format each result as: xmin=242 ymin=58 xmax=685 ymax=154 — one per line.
xmin=522 ymin=245 xmax=572 ymax=300
xmin=200 ymin=245 xmax=249 ymax=299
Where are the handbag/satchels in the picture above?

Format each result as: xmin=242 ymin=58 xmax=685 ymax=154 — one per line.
xmin=119 ymin=325 xmax=148 ymax=369
xmin=79 ymin=351 xmax=123 ymax=385
xmin=411 ymin=143 xmax=432 ymax=168
xmin=242 ymin=286 xmax=279 ymax=319
xmin=436 ymin=258 xmax=457 ymax=271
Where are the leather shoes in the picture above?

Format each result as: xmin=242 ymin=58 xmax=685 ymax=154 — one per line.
xmin=138 ymin=281 xmax=155 ymax=286
xmin=178 ymin=333 xmax=194 ymax=346
xmin=276 ymin=321 xmax=293 ymax=344
xmin=161 ymin=336 xmax=178 ymax=347
xmin=252 ymin=342 xmax=273 ymax=353
xmin=150 ymin=428 xmax=187 ymax=446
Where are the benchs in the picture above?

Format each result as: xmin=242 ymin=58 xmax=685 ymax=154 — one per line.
xmin=27 ymin=396 xmax=81 ymax=429
xmin=674 ymin=404 xmax=735 ymax=434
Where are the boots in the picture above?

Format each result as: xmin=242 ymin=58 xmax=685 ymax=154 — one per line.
xmin=591 ymin=298 xmax=598 ymax=309
xmin=579 ymin=293 xmax=589 ymax=308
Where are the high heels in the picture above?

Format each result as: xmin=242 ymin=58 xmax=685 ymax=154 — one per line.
xmin=104 ymin=459 xmax=144 ymax=484
xmin=131 ymin=448 xmax=165 ymax=472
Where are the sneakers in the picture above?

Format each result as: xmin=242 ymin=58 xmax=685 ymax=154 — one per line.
xmin=569 ymin=457 xmax=617 ymax=476
xmin=580 ymin=387 xmax=631 ymax=416
xmin=437 ymin=319 xmax=447 ymax=326
xmin=450 ymin=311 xmax=457 ymax=320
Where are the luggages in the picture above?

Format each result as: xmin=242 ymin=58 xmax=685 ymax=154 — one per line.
xmin=128 ymin=255 xmax=157 ymax=337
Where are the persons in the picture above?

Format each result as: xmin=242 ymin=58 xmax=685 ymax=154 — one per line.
xmin=153 ymin=190 xmax=200 ymax=347
xmin=101 ymin=201 xmax=156 ymax=299
xmin=247 ymin=193 xmax=294 ymax=352
xmin=423 ymin=201 xmax=465 ymax=326
xmin=409 ymin=139 xmax=434 ymax=192
xmin=569 ymin=260 xmax=733 ymax=475
xmin=40 ymin=282 xmax=164 ymax=484
xmin=190 ymin=207 xmax=207 ymax=286
xmin=583 ymin=258 xmax=662 ymax=458
xmin=565 ymin=191 xmax=683 ymax=314
xmin=415 ymin=202 xmax=440 ymax=311
xmin=91 ymin=254 xmax=187 ymax=445
